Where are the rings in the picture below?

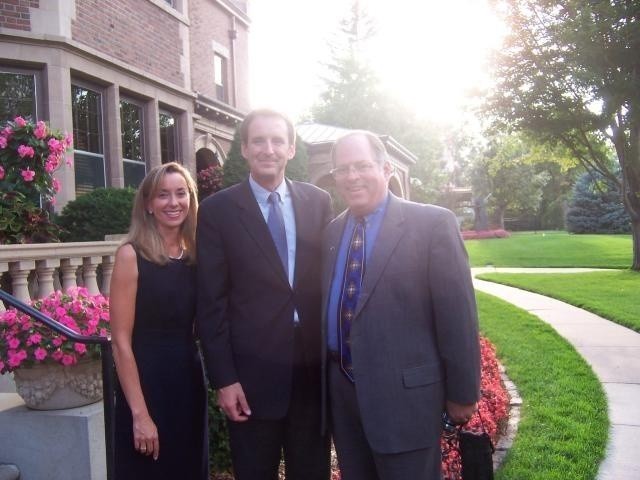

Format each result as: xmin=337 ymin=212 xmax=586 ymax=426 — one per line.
xmin=141 ymin=447 xmax=146 ymax=450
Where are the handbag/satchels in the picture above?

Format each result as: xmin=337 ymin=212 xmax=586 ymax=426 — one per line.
xmin=460 ymin=410 xmax=494 ymax=480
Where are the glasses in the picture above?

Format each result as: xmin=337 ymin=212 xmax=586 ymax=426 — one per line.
xmin=328 ymin=161 xmax=377 ymax=178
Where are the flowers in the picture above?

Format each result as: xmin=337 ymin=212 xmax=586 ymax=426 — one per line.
xmin=0 ymin=285 xmax=113 ymax=374
xmin=0 ymin=118 xmax=75 ymax=240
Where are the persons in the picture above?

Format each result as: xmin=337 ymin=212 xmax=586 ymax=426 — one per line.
xmin=109 ymin=162 xmax=211 ymax=480
xmin=195 ymin=107 xmax=334 ymax=480
xmin=319 ymin=128 xmax=482 ymax=480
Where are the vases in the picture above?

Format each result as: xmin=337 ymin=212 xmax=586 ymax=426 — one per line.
xmin=7 ymin=357 xmax=105 ymax=412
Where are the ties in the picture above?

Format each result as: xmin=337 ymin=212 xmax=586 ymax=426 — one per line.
xmin=267 ymin=192 xmax=288 ymax=282
xmin=337 ymin=217 xmax=366 ymax=383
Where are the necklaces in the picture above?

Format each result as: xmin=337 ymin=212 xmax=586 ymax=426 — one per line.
xmin=170 ymin=250 xmax=183 ymax=260
